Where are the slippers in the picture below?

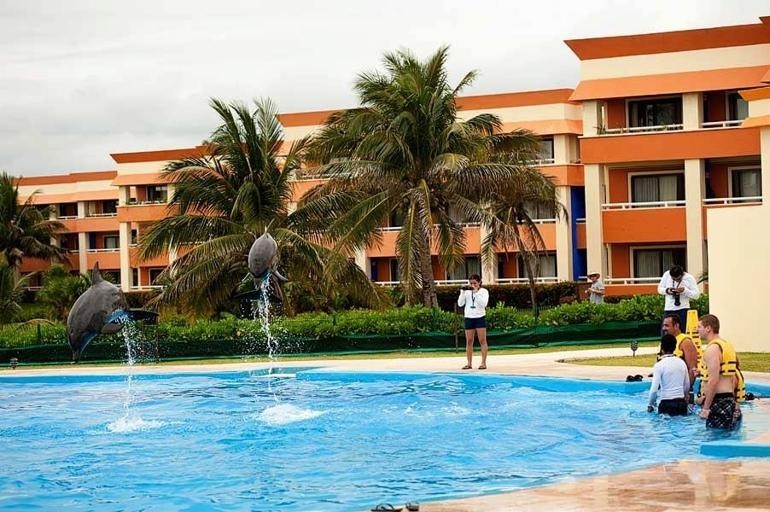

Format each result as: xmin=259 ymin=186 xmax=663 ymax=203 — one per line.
xmin=371 ymin=500 xmax=420 ymax=512
xmin=625 ymin=375 xmax=644 ymax=382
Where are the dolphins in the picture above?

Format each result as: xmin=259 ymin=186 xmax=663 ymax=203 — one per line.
xmin=247 ymin=218 xmax=288 ymax=302
xmin=66 ymin=261 xmax=159 ymax=363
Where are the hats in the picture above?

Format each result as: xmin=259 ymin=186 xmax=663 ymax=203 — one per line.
xmin=587 ymin=272 xmax=600 ymax=280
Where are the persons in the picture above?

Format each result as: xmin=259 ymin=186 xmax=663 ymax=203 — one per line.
xmin=457 ymin=274 xmax=489 ymax=369
xmin=648 ymin=333 xmax=690 ymax=417
xmin=662 ymin=313 xmax=698 ymax=393
xmin=734 ymin=356 xmax=746 ymax=421
xmin=584 ymin=272 xmax=605 ymax=305
xmin=696 ymin=314 xmax=737 ymax=428
xmin=657 ymin=265 xmax=699 ymax=356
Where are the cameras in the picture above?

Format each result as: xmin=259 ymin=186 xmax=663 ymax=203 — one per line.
xmin=463 ymin=285 xmax=474 ymax=290
xmin=670 ymin=287 xmax=682 ymax=307
